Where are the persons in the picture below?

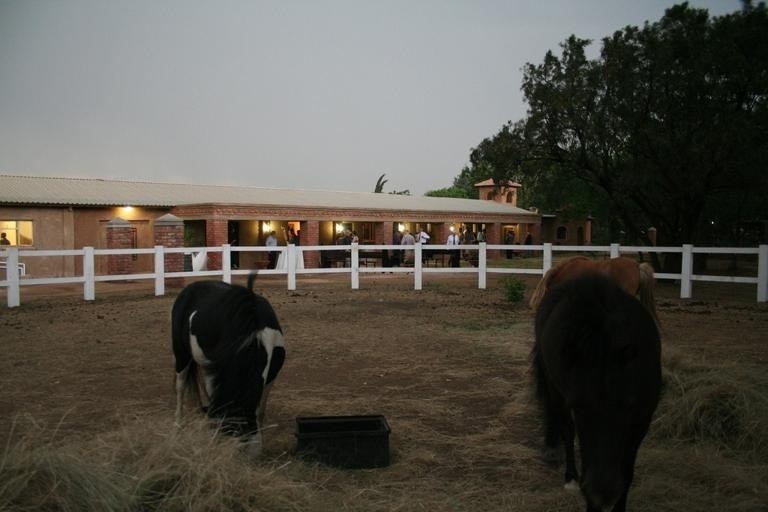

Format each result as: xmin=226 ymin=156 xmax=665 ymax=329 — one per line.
xmin=349 ymin=231 xmax=360 ymax=246
xmin=0 ymin=232 xmax=10 ymax=245
xmin=265 ymin=230 xmax=280 ymax=269
xmin=504 ymin=231 xmax=515 ymax=262
xmin=335 ymin=232 xmax=349 ymax=245
xmin=523 ymin=232 xmax=533 ymax=255
xmin=457 ymin=226 xmax=487 ymax=259
xmin=445 ymin=230 xmax=460 ymax=268
xmin=399 ymin=230 xmax=416 ymax=264
xmin=414 ymin=228 xmax=431 ymax=265
xmin=287 ymin=228 xmax=301 ymax=246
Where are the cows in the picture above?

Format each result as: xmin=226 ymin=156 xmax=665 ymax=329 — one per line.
xmin=533 ymin=254 xmax=664 ymax=511
xmin=168 ymin=272 xmax=287 ymax=443
